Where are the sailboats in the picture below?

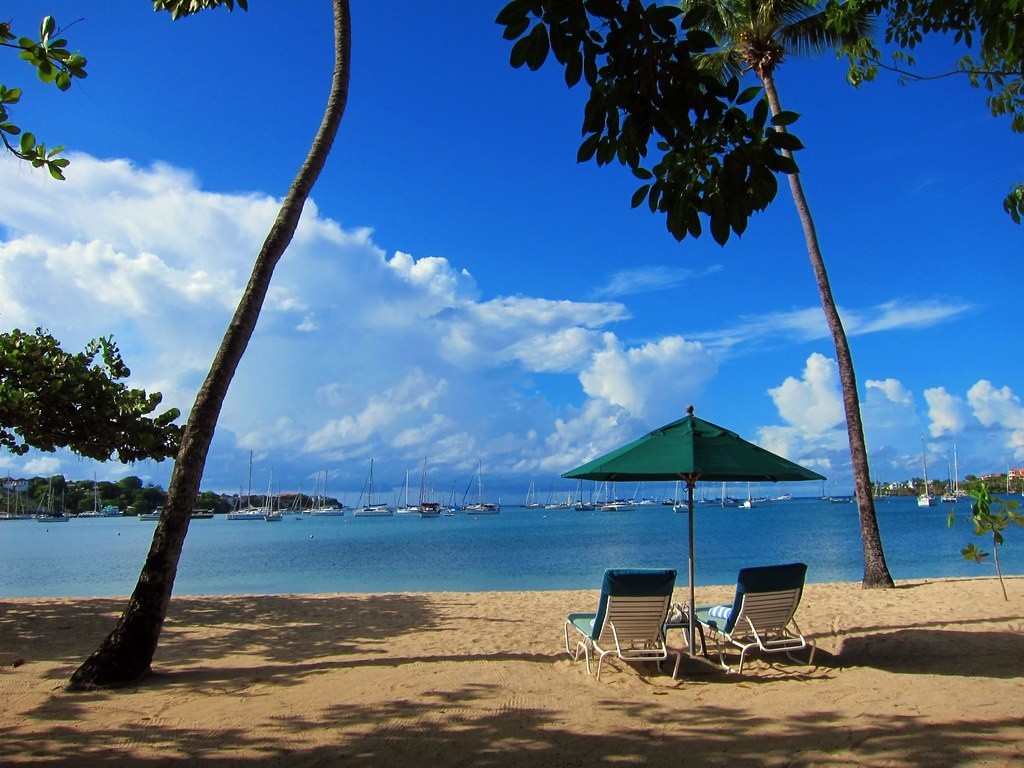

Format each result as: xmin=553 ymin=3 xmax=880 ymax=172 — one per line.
xmin=354 ymin=459 xmax=393 ymax=516
xmin=520 ymin=480 xmax=539 ymax=508
xmin=226 ymin=450 xmax=269 ymax=519
xmin=417 ymin=457 xmax=442 ymax=518
xmin=917 ymin=436 xmax=937 ymax=506
xmin=0 ymin=468 xmax=33 ymax=519
xmin=303 ymin=470 xmax=344 ymax=516
xmin=78 ymin=471 xmax=103 ymax=518
xmin=264 ymin=466 xmax=283 ymax=522
xmin=465 ymin=460 xmax=501 ymax=514
xmin=37 ymin=479 xmax=69 ymax=522
xmin=574 ymin=479 xmax=595 ymax=510
xmin=396 ymin=470 xmax=412 ymax=513
xmin=595 ymin=481 xmax=638 ymax=511
xmin=940 ymin=451 xmax=958 ymax=502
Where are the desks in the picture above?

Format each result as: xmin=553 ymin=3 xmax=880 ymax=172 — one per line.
xmin=662 ymin=621 xmax=707 ymax=662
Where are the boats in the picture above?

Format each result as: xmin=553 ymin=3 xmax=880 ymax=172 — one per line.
xmin=673 ymin=503 xmax=689 ymax=514
xmin=138 ymin=506 xmax=214 ymax=521
xmin=101 ymin=505 xmax=124 ymax=517
xmin=545 ymin=503 xmax=571 ymax=509
xmin=661 ymin=498 xmax=679 ymax=505
xmin=632 ymin=498 xmax=659 ymax=507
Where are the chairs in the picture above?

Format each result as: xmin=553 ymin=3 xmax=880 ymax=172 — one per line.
xmin=564 ymin=569 xmax=681 ymax=681
xmin=682 ymin=563 xmax=815 ymax=674
